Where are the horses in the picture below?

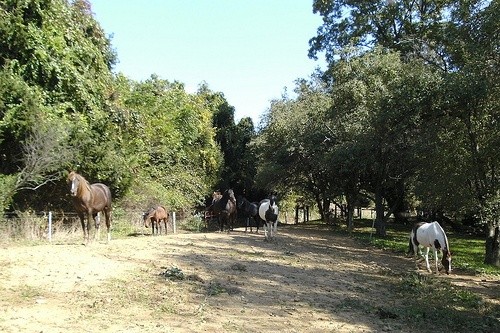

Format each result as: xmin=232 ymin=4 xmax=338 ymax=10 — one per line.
xmin=211 ymin=191 xmax=223 ymax=231
xmin=217 ymin=187 xmax=236 ymax=232
xmin=258 ymin=191 xmax=279 ymax=240
xmin=67 ymin=171 xmax=112 ymax=245
xmin=405 ymin=221 xmax=451 ymax=275
xmin=237 ymin=194 xmax=259 ymax=234
xmin=142 ymin=206 xmax=170 ymax=235
